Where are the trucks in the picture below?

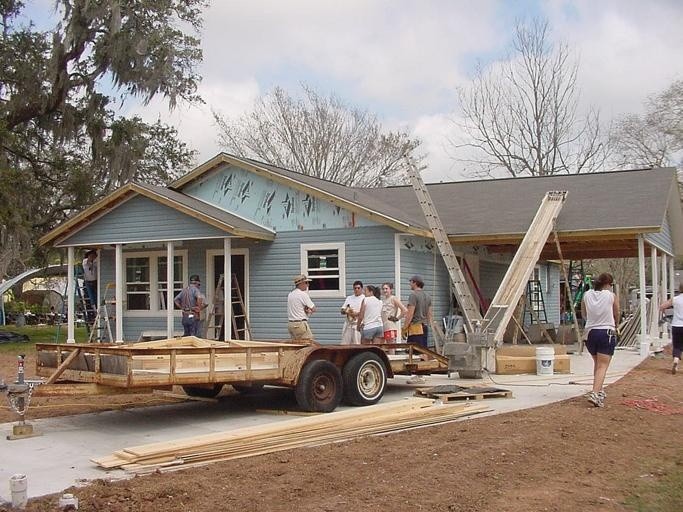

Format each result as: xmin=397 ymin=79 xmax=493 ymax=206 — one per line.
xmin=33 ymin=335 xmax=452 ymax=413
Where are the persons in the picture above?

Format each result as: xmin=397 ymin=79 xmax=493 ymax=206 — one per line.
xmin=174 ymin=274 xmax=203 ymax=337
xmin=381 ymin=282 xmax=408 ymax=355
xmin=580 ymin=271 xmax=621 ymax=409
xmin=400 ymin=275 xmax=435 ymax=384
xmin=357 ymin=285 xmax=384 ymax=344
xmin=340 ymin=280 xmax=366 ymax=345
xmin=659 ymin=282 xmax=683 ymax=374
xmin=83 ymin=249 xmax=98 ymax=312
xmin=287 ymin=274 xmax=316 ymax=339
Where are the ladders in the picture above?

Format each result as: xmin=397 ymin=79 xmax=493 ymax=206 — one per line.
xmin=522 ymin=281 xmax=547 ymax=326
xmin=86 ymin=282 xmax=125 ymax=343
xmin=203 ymin=273 xmax=252 ymax=341
xmin=55 ymin=263 xmax=98 ymax=343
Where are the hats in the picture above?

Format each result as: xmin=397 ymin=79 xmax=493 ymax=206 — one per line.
xmin=408 ymin=276 xmax=423 ymax=283
xmin=291 ymin=274 xmax=313 ymax=286
xmin=190 ymin=274 xmax=200 ymax=281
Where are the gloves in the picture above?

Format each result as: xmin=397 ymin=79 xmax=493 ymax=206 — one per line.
xmin=388 ymin=316 xmax=398 ymax=321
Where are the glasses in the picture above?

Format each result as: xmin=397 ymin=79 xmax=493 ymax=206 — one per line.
xmin=353 ymin=287 xmax=361 ymax=289
xmin=608 ymin=283 xmax=614 ymax=286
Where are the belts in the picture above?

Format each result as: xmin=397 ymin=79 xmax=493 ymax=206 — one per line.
xmin=289 ymin=319 xmax=307 ymax=322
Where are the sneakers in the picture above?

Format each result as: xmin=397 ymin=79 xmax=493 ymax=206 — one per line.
xmin=584 ymin=391 xmax=606 ymax=399
xmin=588 ymin=395 xmax=606 ymax=408
xmin=672 ymin=360 xmax=678 ymax=374
